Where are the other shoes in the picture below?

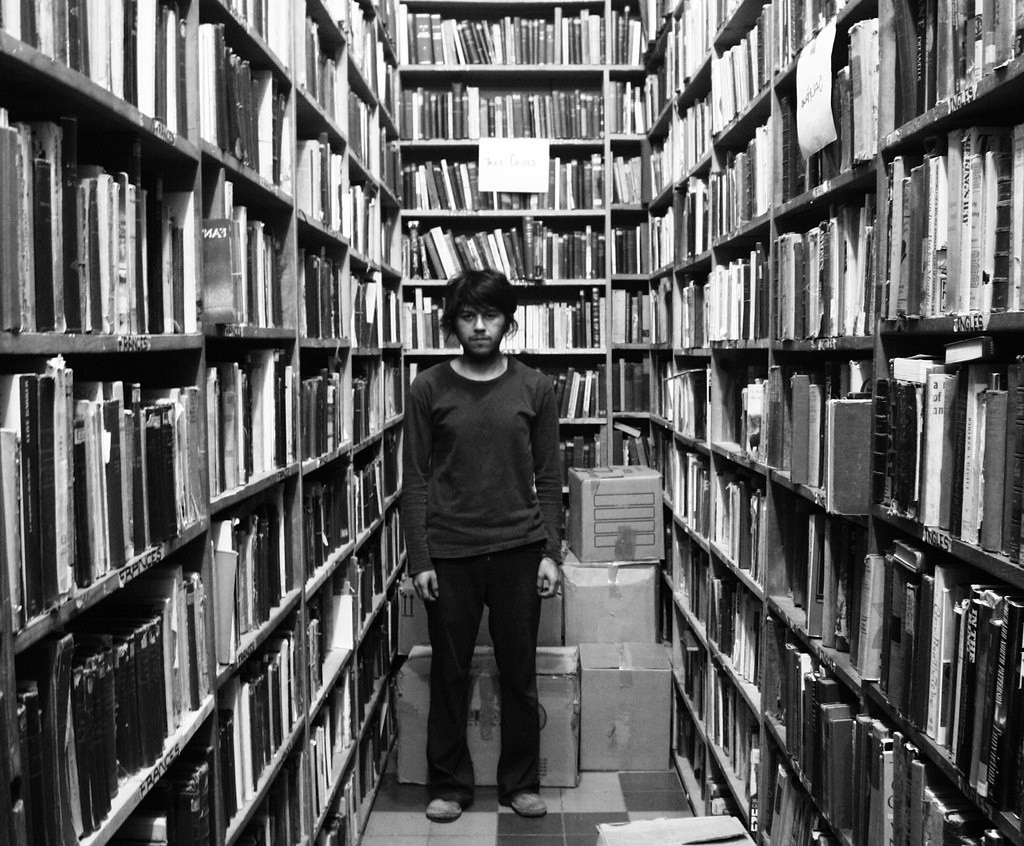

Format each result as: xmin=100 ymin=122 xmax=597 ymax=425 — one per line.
xmin=511 ymin=792 xmax=547 ymax=816
xmin=426 ymin=798 xmax=462 ymax=819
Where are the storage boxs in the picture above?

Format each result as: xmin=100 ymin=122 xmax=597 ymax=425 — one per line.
xmin=579 ymin=643 xmax=673 ymax=771
xmin=562 ymin=540 xmax=658 ymax=646
xmin=395 ymin=644 xmax=580 ymax=786
xmin=595 ymin=816 xmax=757 ymax=846
xmin=568 ymin=464 xmax=664 ymax=563
xmin=397 ymin=577 xmax=562 ymax=655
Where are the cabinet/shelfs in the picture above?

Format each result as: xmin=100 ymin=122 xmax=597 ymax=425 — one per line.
xmin=1 ymin=0 xmax=1024 ymax=846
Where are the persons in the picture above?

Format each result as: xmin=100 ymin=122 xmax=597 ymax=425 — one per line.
xmin=400 ymin=270 xmax=564 ymax=819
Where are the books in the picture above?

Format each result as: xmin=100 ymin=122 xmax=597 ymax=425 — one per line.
xmin=1 ymin=0 xmax=1024 ymax=845
